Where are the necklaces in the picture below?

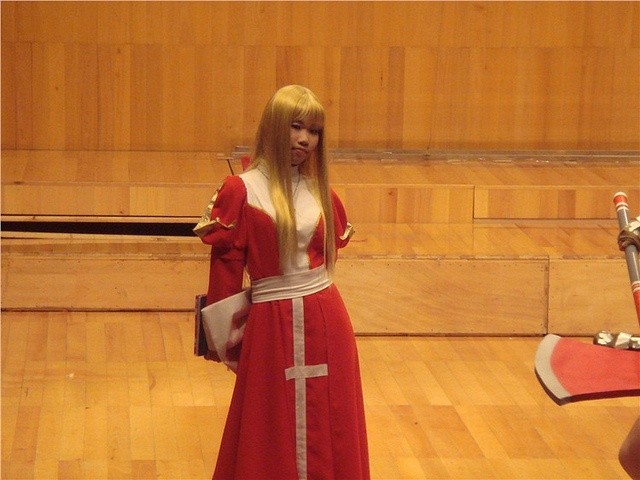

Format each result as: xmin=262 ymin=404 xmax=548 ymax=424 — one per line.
xmin=257 ymin=165 xmax=300 ymax=212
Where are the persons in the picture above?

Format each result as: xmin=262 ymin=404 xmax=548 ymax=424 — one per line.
xmin=196 ymin=83 xmax=372 ymax=480
